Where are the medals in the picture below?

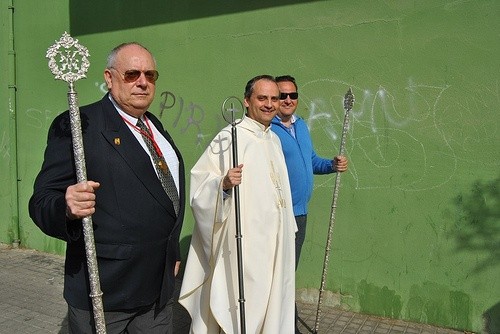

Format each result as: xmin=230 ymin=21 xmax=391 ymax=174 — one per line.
xmin=158 ymin=159 xmax=168 ymax=174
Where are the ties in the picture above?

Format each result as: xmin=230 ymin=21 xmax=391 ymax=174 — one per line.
xmin=136 ymin=117 xmax=180 ymax=218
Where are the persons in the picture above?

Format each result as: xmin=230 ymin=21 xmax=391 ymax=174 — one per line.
xmin=270 ymin=75 xmax=348 ymax=334
xmin=178 ymin=75 xmax=298 ymax=334
xmin=28 ymin=40 xmax=185 ymax=334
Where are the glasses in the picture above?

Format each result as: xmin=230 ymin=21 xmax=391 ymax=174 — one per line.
xmin=109 ymin=66 xmax=160 ymax=83
xmin=280 ymin=92 xmax=299 ymax=100
